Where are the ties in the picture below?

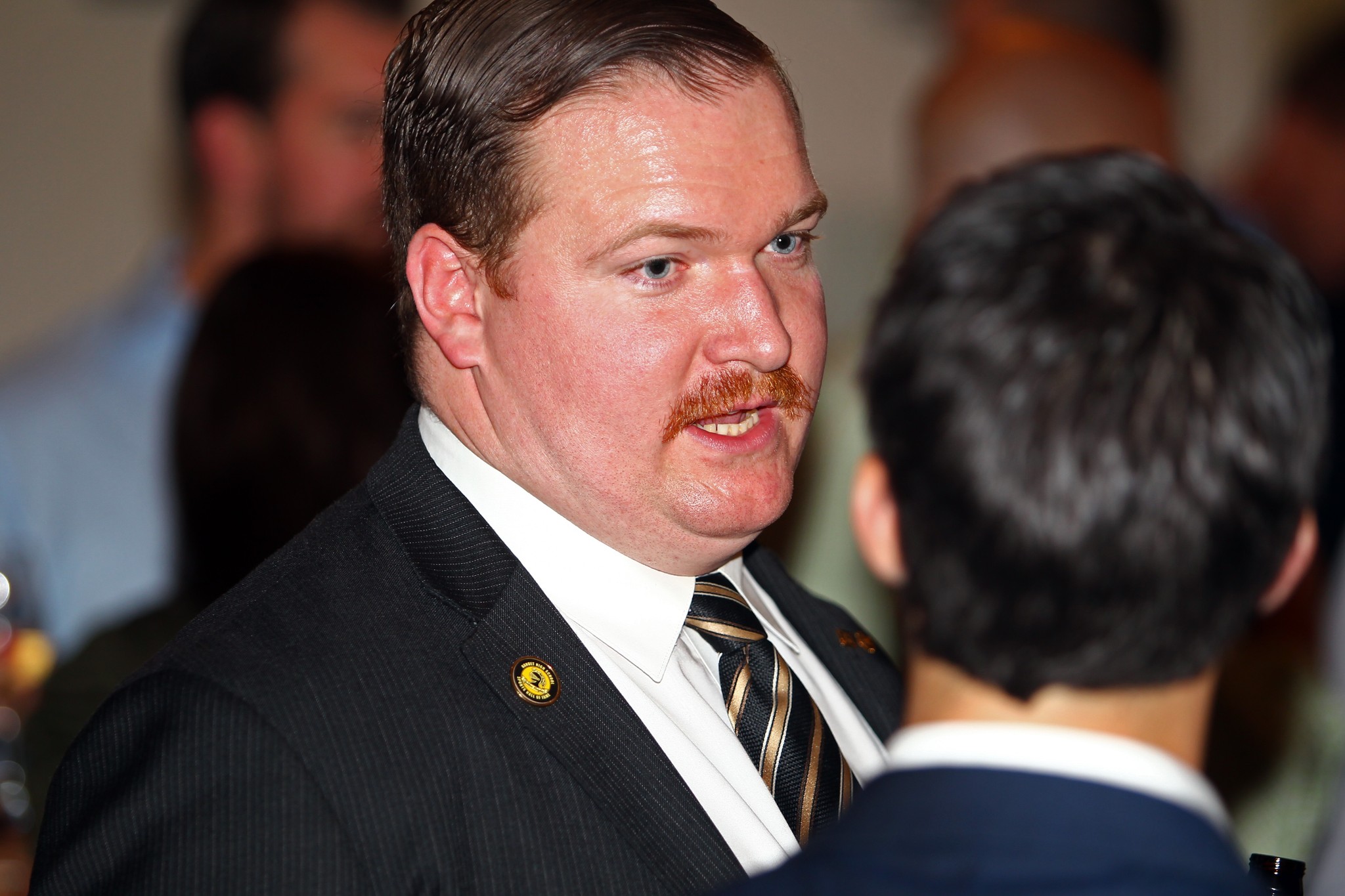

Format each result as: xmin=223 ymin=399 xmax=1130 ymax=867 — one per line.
xmin=684 ymin=569 xmax=862 ymax=854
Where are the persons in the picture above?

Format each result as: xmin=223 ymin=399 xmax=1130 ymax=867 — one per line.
xmin=2 ymin=0 xmax=416 ymax=662
xmin=889 ymin=18 xmax=1184 ymax=248
xmin=693 ymin=141 xmax=1332 ymax=894
xmin=26 ymin=1 xmax=902 ymax=894
xmin=11 ymin=241 xmax=418 ymax=848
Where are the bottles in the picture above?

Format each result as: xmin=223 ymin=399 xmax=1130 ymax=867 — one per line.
xmin=1251 ymin=854 xmax=1306 ymax=895
